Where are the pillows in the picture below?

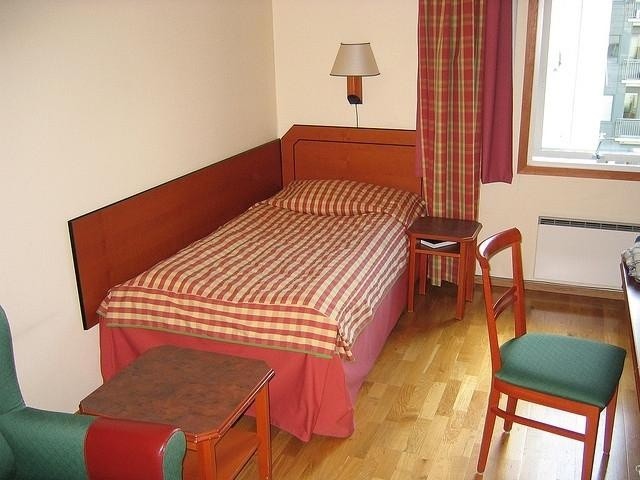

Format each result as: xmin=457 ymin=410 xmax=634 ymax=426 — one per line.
xmin=268 ymin=178 xmax=425 ymax=230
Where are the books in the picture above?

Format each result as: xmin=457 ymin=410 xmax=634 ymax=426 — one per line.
xmin=420 ymin=240 xmax=457 ymax=249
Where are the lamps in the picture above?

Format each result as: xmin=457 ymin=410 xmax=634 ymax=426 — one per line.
xmin=330 ymin=42 xmax=380 ymax=104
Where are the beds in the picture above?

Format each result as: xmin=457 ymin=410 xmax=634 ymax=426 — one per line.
xmin=96 ymin=124 xmax=426 ymax=444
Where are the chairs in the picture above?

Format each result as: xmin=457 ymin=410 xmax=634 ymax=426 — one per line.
xmin=474 ymin=228 xmax=627 ymax=480
xmin=0 ymin=306 xmax=187 ymax=480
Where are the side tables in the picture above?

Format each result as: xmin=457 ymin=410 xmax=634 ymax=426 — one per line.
xmin=406 ymin=216 xmax=483 ymax=321
xmin=79 ymin=344 xmax=275 ymax=480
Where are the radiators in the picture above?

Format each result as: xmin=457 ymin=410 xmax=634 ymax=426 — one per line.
xmin=532 ymin=215 xmax=640 ymax=291
